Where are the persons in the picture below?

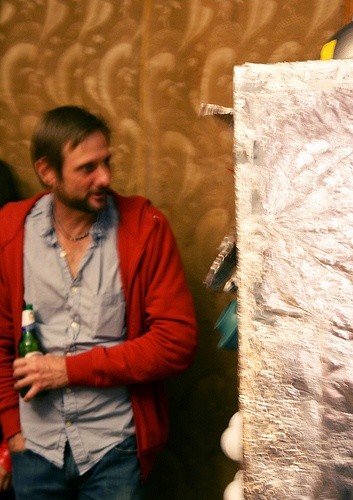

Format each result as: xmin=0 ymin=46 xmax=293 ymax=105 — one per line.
xmin=1 ymin=105 xmax=200 ymax=500
xmin=1 ymin=161 xmax=28 ymax=498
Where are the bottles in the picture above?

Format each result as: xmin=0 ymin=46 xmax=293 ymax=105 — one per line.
xmin=17 ymin=304 xmax=50 ymax=400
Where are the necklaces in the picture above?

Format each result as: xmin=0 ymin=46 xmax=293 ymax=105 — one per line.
xmin=48 ymin=201 xmax=94 ymax=240
